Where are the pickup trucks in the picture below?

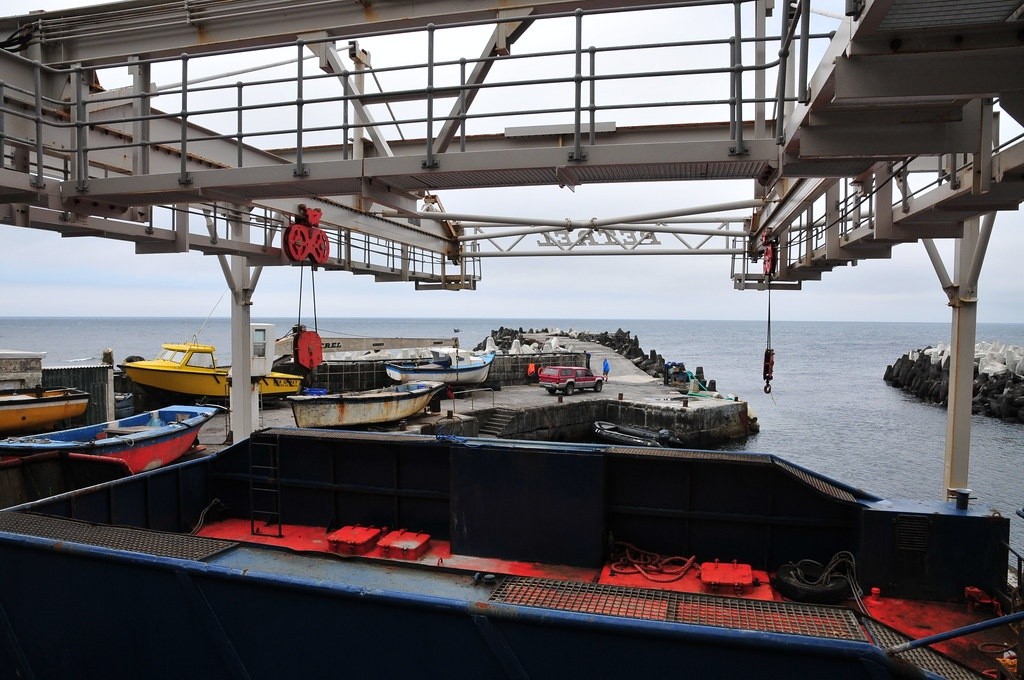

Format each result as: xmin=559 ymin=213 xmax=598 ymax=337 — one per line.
xmin=539 ymin=366 xmax=604 ymax=396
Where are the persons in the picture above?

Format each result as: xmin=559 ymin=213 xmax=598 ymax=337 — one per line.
xmin=603 ymin=358 xmax=609 ymax=381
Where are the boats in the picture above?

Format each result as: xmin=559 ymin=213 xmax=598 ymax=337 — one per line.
xmin=593 ymin=421 xmax=685 ymax=450
xmin=116 ymin=340 xmax=304 ymax=400
xmin=288 ymin=379 xmax=446 ymax=428
xmin=0 ymin=404 xmax=220 ymax=510
xmin=0 ymin=384 xmax=91 ymax=434
xmin=384 ymin=351 xmax=497 ymax=384
